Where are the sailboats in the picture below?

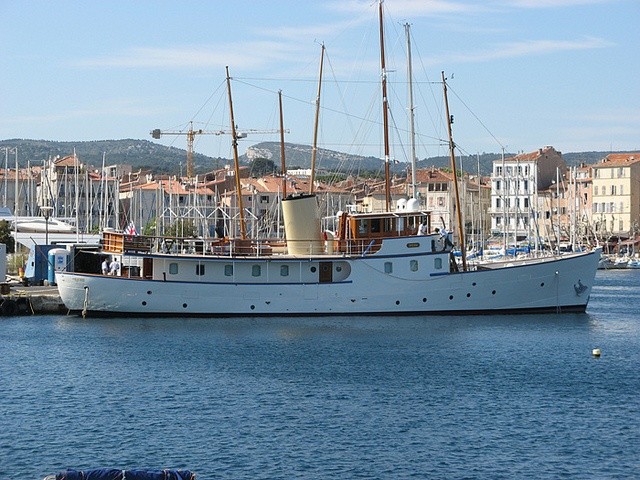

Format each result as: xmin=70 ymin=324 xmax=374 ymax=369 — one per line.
xmin=52 ymin=0 xmax=602 ymax=314
xmin=459 ymin=148 xmax=639 ymax=270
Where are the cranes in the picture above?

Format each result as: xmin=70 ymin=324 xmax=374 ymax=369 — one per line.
xmin=148 ymin=120 xmax=291 ymax=179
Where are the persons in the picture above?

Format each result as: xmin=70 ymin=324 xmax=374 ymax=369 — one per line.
xmin=110 ymin=258 xmax=119 ymax=276
xmin=435 ymin=228 xmax=454 ymax=251
xmin=102 ymin=258 xmax=108 ymax=275
xmin=417 ymin=220 xmax=427 ymax=236
